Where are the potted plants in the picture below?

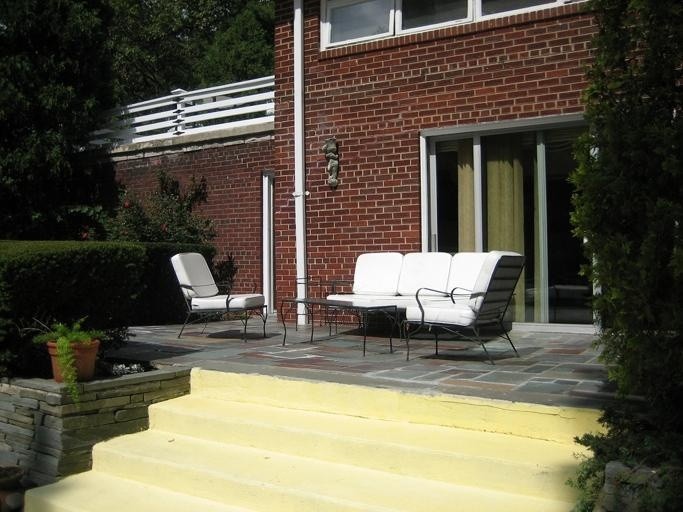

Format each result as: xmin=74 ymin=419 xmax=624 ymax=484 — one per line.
xmin=33 ymin=315 xmax=106 ymax=410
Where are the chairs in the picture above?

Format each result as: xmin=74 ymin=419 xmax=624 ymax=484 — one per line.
xmin=325 ymin=251 xmax=527 ymax=365
xmin=169 ymin=252 xmax=267 ymax=343
